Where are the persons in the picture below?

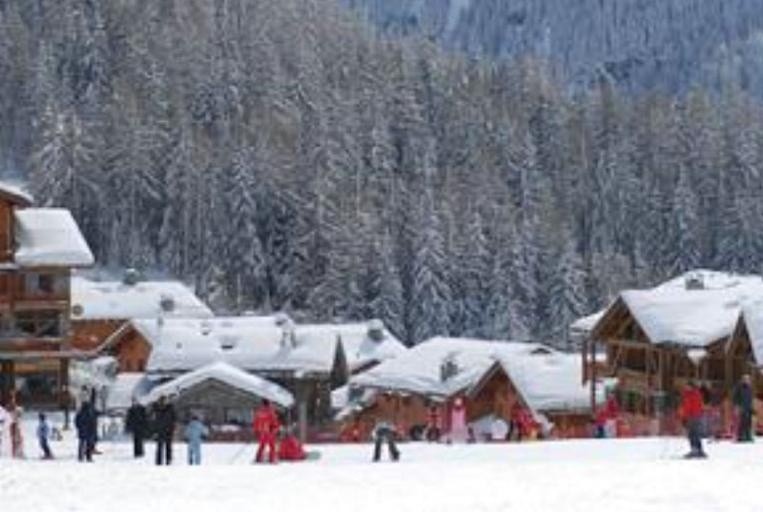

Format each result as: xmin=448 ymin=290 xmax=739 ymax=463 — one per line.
xmin=150 ymin=394 xmax=176 ymax=466
xmin=730 ymin=373 xmax=754 ymax=442
xmin=100 ymin=384 xmax=107 ymax=411
xmin=677 ymin=381 xmax=710 ymax=458
xmin=250 ymin=397 xmax=278 ymax=462
xmin=371 ymin=419 xmax=401 ymax=461
xmin=77 ymin=385 xmax=88 ymax=403
xmin=35 ymin=411 xmax=53 ymax=460
xmin=182 ymin=414 xmax=208 ymax=466
xmin=122 ymin=393 xmax=148 ymax=457
xmin=72 ymin=399 xmax=94 ymax=462
xmin=90 ymin=386 xmax=96 ymax=405
xmin=450 ymin=396 xmax=467 ymax=448
xmin=502 ymin=398 xmax=526 ymax=442
